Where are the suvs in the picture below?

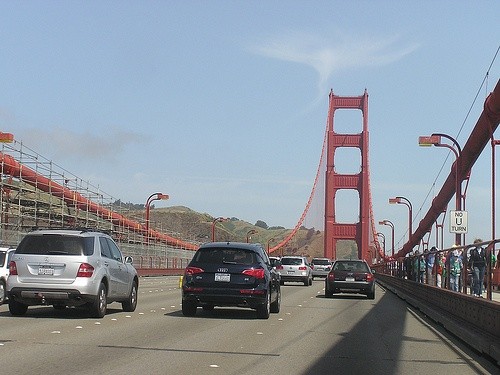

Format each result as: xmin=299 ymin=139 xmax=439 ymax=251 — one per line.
xmin=309 ymin=257 xmax=334 ymax=279
xmin=5 ymin=226 xmax=139 ymax=319
xmin=181 ymin=242 xmax=284 ymax=319
xmin=279 ymin=255 xmax=314 ymax=286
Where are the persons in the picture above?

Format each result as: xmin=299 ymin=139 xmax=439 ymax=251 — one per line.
xmin=377 ymin=239 xmax=500 ymax=299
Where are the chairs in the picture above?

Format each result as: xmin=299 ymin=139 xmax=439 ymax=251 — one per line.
xmin=355 ymin=263 xmax=362 ymax=270
xmin=338 ymin=263 xmax=345 ymax=269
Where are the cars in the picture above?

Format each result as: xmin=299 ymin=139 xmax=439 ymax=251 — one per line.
xmin=325 ymin=259 xmax=377 ymax=300
xmin=0 ymin=247 xmax=16 ymax=305
xmin=268 ymin=257 xmax=281 ymax=274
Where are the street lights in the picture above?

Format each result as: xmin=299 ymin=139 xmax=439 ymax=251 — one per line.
xmin=379 ymin=219 xmax=394 ymax=257
xmin=145 ymin=192 xmax=170 ymax=245
xmin=376 ymin=232 xmax=385 ymax=256
xmin=418 ymin=132 xmax=461 ymax=247
xmin=388 ymin=196 xmax=412 ymax=240
xmin=212 ymin=216 xmax=230 ymax=242
xmin=247 ymin=229 xmax=258 ymax=243
xmin=267 ymin=237 xmax=277 ymax=257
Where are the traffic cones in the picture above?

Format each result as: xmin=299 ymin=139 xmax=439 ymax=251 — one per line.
xmin=177 ymin=276 xmax=183 ymax=289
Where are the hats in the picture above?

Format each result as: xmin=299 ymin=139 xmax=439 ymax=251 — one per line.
xmin=452 ymin=244 xmax=457 ymax=247
xmin=430 ymin=247 xmax=437 ymax=251
